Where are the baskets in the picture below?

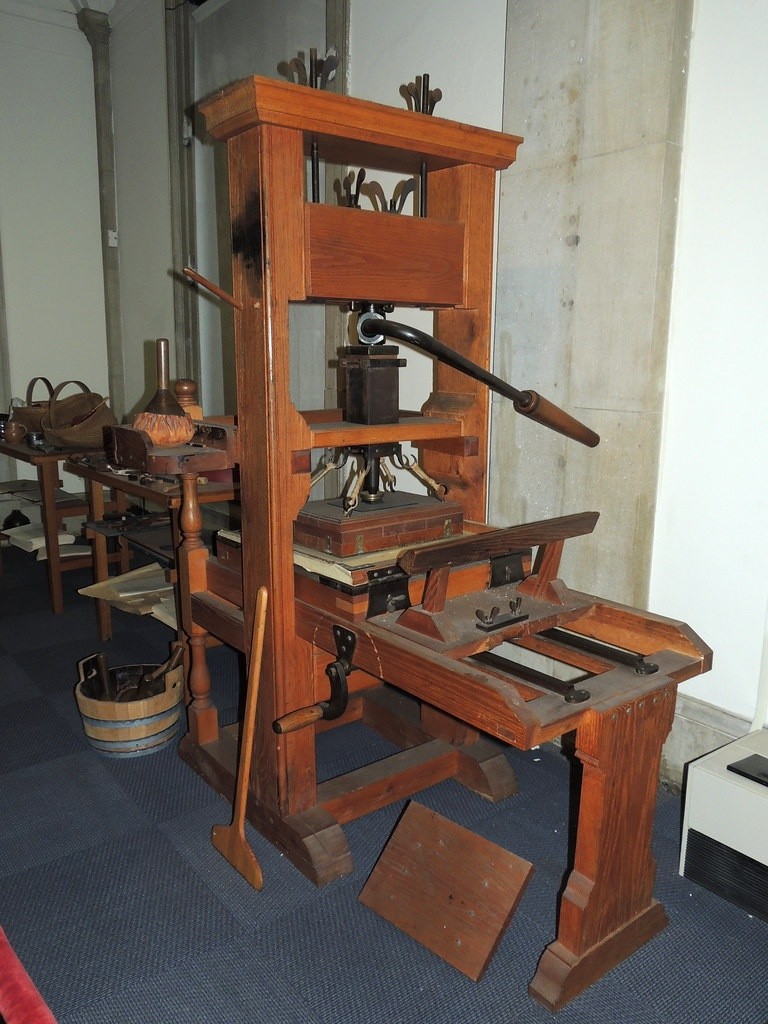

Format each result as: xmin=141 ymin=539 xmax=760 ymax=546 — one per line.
xmin=41 ymin=380 xmax=117 ymax=448
xmin=12 ymin=377 xmax=66 ymax=434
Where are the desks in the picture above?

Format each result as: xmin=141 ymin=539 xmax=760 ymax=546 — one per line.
xmin=65 ymin=462 xmax=243 ymax=705
xmin=0 ymin=439 xmax=104 ymax=617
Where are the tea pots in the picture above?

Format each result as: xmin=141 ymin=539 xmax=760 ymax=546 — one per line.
xmin=4 ymin=419 xmax=27 ymax=444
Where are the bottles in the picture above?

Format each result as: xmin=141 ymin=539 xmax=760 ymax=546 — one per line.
xmin=3 ymin=509 xmax=30 ymax=530
xmin=133 ymin=339 xmax=195 ymax=447
